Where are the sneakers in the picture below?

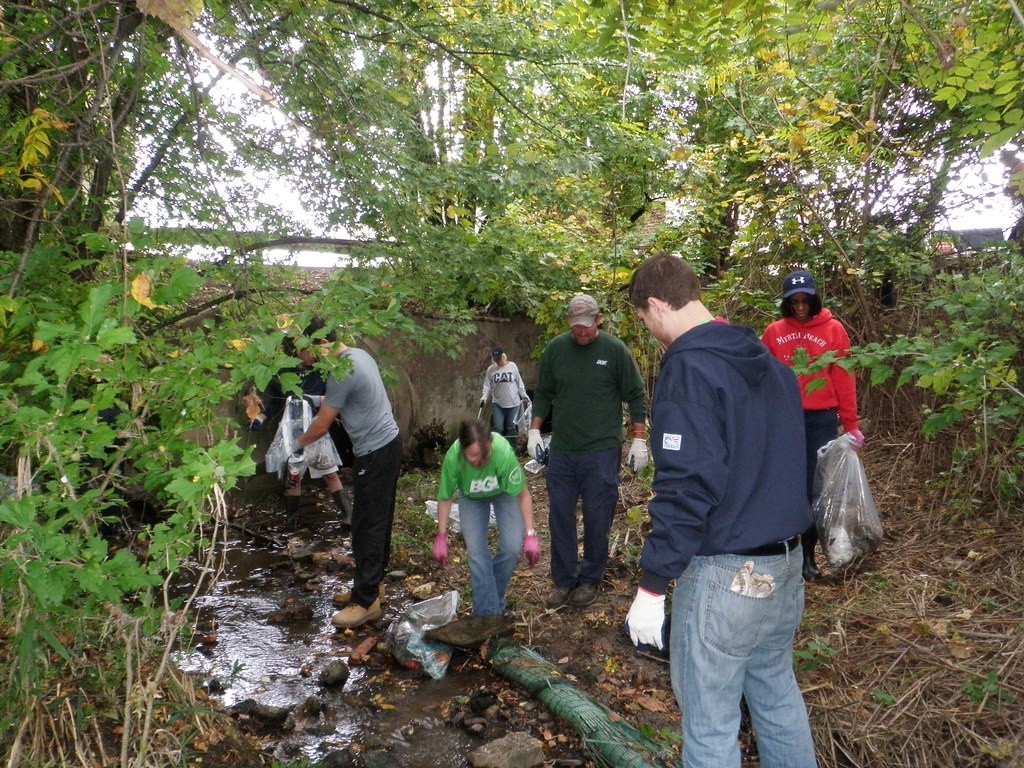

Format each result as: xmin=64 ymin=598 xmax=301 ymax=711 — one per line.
xmin=331 ymin=598 xmax=382 ymax=629
xmin=332 ymin=584 xmax=386 ymax=606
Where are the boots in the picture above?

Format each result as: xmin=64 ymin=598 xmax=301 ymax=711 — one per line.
xmin=801 ymin=530 xmax=822 ymax=581
xmin=332 ymin=489 xmax=353 ymax=526
xmin=277 ymin=495 xmax=302 ymax=533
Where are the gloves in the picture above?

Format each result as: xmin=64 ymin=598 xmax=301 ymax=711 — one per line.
xmin=291 ymin=395 xmax=321 ymax=408
xmin=432 ymin=532 xmax=449 ymax=566
xmin=522 ymin=396 xmax=530 ymax=404
xmin=527 ymin=429 xmax=545 ymax=464
xmin=479 ymin=397 xmax=487 ymax=407
xmin=625 ymin=586 xmax=666 ymax=651
xmin=626 ymin=438 xmax=649 ymax=473
xmin=524 ymin=536 xmax=541 ymax=566
xmin=245 ymin=418 xmax=261 ymax=433
xmin=847 ymin=430 xmax=866 ymax=451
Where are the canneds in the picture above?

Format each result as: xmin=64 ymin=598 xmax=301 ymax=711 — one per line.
xmin=289 ymin=467 xmax=300 ymax=482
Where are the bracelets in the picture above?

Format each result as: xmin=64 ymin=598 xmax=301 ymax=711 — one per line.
xmin=631 ymin=430 xmax=647 ymax=436
xmin=527 ymin=530 xmax=537 ymax=536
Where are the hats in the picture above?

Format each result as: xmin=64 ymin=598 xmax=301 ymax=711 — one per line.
xmin=568 ymin=295 xmax=599 ymax=329
xmin=782 ymin=271 xmax=816 ymax=297
xmin=492 ymin=348 xmax=503 ymax=361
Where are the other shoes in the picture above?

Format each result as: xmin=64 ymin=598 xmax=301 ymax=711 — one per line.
xmin=572 ymin=583 xmax=600 ymax=607
xmin=546 ymin=582 xmax=578 ymax=607
xmin=501 ymin=609 xmax=519 ymax=623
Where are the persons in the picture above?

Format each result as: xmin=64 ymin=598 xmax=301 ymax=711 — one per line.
xmin=479 ymin=348 xmax=530 ymax=445
xmin=527 ymin=294 xmax=648 ymax=610
xmin=248 ymin=336 xmax=352 ymax=528
xmin=432 ymin=420 xmax=540 ymax=616
xmin=283 ymin=318 xmax=404 ymax=628
xmin=761 ymin=272 xmax=864 ymax=581
xmin=625 ymin=253 xmax=818 ymax=768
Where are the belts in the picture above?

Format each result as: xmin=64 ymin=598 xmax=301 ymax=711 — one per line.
xmin=737 ymin=534 xmax=799 ymax=556
